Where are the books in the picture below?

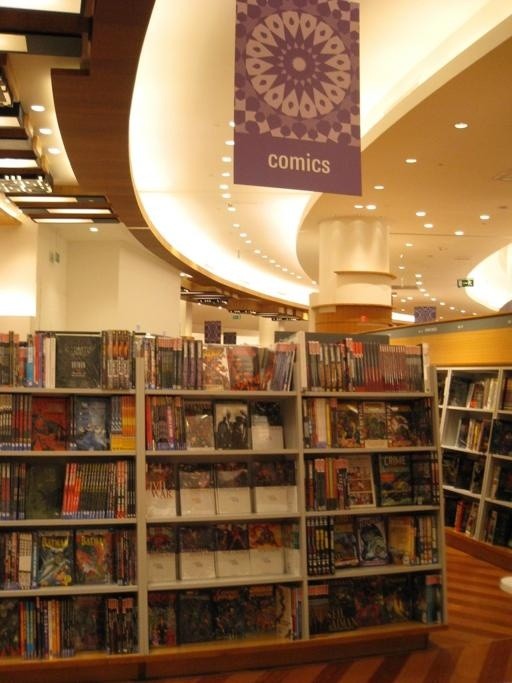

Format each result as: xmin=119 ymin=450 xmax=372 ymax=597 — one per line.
xmin=309 ymin=337 xmax=430 ymax=393
xmin=146 ymin=463 xmax=298 ymax=517
xmin=0 ymin=330 xmax=135 ymax=389
xmin=0 ymin=529 xmax=137 ymax=590
xmin=305 ymin=453 xmax=435 ymax=510
xmin=0 ymin=460 xmax=137 ymax=520
xmin=146 ymin=395 xmax=283 ymax=449
xmin=0 ymin=595 xmax=136 ymax=661
xmin=148 ymin=583 xmax=301 ymax=648
xmin=304 ymin=398 xmax=433 ymax=448
xmin=0 ymin=394 xmax=137 ymax=450
xmin=305 ymin=515 xmax=439 ymax=575
xmin=310 ymin=571 xmax=442 ymax=634
xmin=435 ymin=368 xmax=512 ymax=551
xmin=147 ymin=524 xmax=299 ymax=582
xmin=144 ymin=337 xmax=294 ymax=391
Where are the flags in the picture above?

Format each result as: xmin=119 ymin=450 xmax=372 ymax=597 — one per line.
xmin=232 ymin=1 xmax=362 ymax=197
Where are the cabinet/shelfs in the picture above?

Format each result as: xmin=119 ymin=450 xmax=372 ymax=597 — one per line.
xmin=0 ymin=327 xmax=452 ymax=681
xmin=431 ymin=361 xmax=511 ymax=576
